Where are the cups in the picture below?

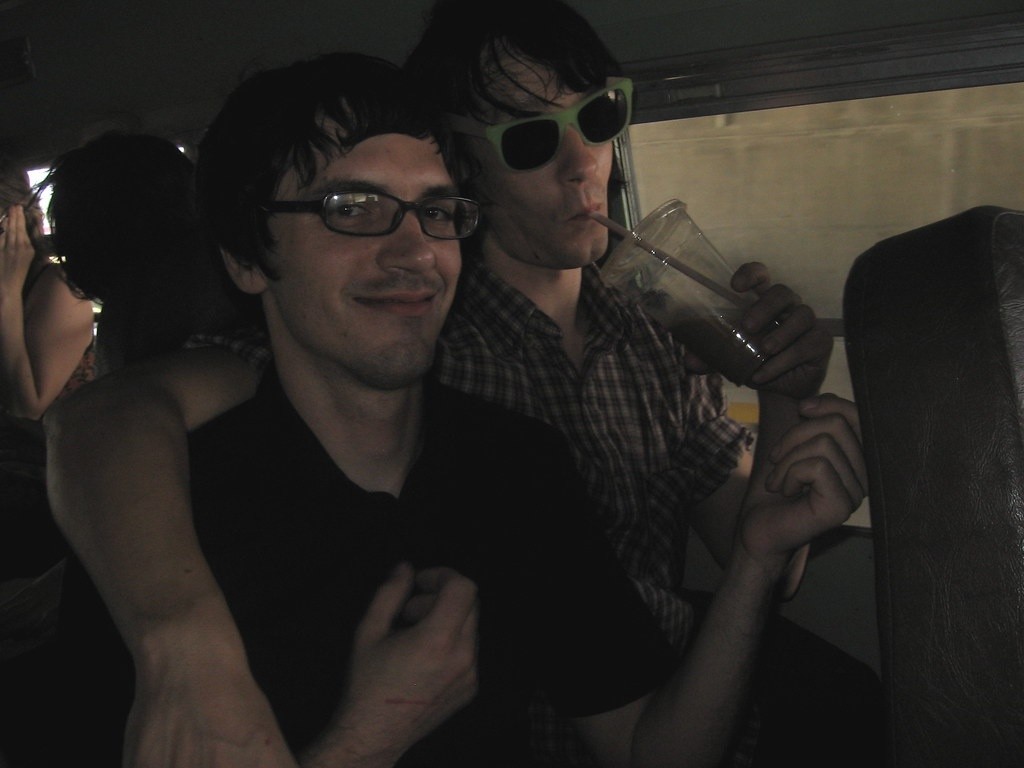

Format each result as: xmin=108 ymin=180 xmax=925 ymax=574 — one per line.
xmin=598 ymin=195 xmax=788 ymax=386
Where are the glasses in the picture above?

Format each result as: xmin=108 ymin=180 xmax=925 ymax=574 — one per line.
xmin=444 ymin=76 xmax=635 ymax=173
xmin=255 ymin=189 xmax=482 ymax=240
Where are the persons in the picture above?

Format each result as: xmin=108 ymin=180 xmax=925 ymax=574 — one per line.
xmin=0 ymin=0 xmax=886 ymax=768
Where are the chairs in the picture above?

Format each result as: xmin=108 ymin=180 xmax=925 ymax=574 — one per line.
xmin=842 ymin=207 xmax=1024 ymax=768
